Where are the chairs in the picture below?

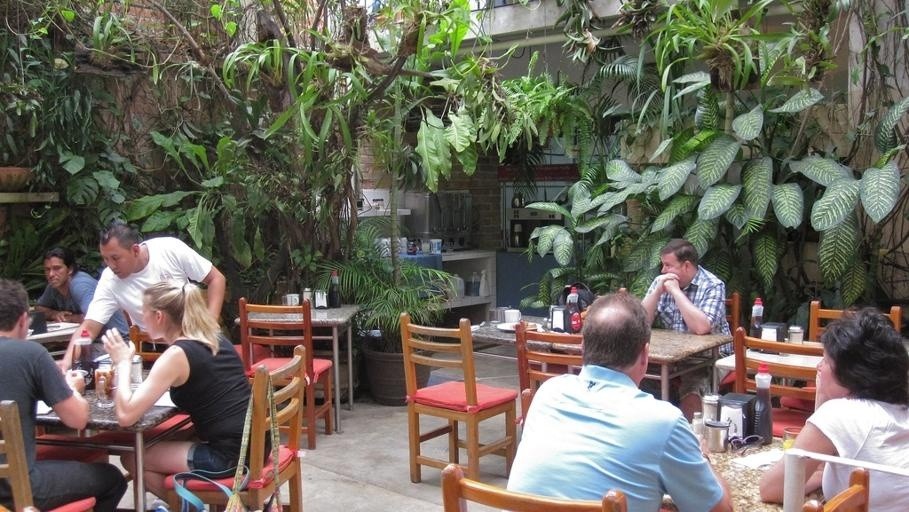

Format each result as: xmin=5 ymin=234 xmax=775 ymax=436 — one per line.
xmin=804 ymin=468 xmax=870 ymax=512
xmin=238 ymin=296 xmax=333 ymax=449
xmin=736 ymin=327 xmax=823 ymax=436
xmin=399 ymin=312 xmax=518 ymax=484
xmin=809 ymin=300 xmax=902 ymax=342
xmin=724 ymin=291 xmax=740 ymax=335
xmin=514 ymin=323 xmax=584 ymax=428
xmin=0 ymin=399 xmax=96 ymax=512
xmin=129 ymin=325 xmax=167 ymax=368
xmin=164 ymin=345 xmax=310 ymax=511
xmin=440 ymin=464 xmax=624 ymax=512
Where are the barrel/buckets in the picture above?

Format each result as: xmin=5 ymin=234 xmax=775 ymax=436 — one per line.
xmin=466 ymin=273 xmax=479 ymax=295
xmin=445 ymin=273 xmax=465 ymax=298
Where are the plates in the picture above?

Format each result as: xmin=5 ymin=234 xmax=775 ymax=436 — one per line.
xmin=497 ymin=322 xmax=541 ymax=331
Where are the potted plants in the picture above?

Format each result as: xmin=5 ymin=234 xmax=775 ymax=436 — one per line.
xmin=1 ymin=48 xmax=40 ymax=189
xmin=358 ymin=252 xmax=455 ymax=405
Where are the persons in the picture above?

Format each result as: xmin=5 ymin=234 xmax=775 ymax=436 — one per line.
xmin=501 ymin=291 xmax=734 ymax=512
xmin=639 ymin=236 xmax=734 ymax=423
xmin=101 ymin=278 xmax=272 ymax=512
xmin=0 ymin=277 xmax=128 ymax=512
xmin=34 ymin=246 xmax=129 ymax=390
xmin=759 ymin=304 xmax=909 ymax=512
xmin=56 ymin=222 xmax=226 ymax=375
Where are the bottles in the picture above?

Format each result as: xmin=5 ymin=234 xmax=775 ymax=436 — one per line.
xmin=302 ymin=288 xmax=313 ymax=309
xmin=704 ymin=419 xmax=730 ymax=452
xmin=750 ymin=297 xmax=764 ymax=352
xmin=692 ymin=412 xmax=703 ymax=434
xmin=328 ymin=271 xmax=341 ymax=308
xmin=567 ymin=286 xmax=582 ymax=334
xmin=702 ymin=395 xmax=720 ymax=438
xmin=314 ymin=289 xmax=328 ymax=309
xmin=753 ymin=363 xmax=773 ymax=444
xmin=72 ymin=330 xmax=95 ymax=389
xmin=407 ymin=239 xmax=416 ymax=255
xmin=399 ymin=238 xmax=407 ymax=254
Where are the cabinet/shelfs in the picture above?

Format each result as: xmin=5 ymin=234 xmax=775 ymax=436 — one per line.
xmin=429 ymin=250 xmax=496 ymax=322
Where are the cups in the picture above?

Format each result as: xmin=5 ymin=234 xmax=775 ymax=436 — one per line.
xmin=95 ymin=369 xmax=119 ymax=408
xmin=782 ymin=426 xmax=802 ymax=453
xmin=505 ymin=310 xmax=521 ymax=322
xmin=30 ymin=311 xmax=48 ymax=335
xmin=421 ymin=240 xmax=430 ymax=254
xmin=286 ymin=294 xmax=300 ymax=305
xmin=430 ymin=239 xmax=442 ymax=254
